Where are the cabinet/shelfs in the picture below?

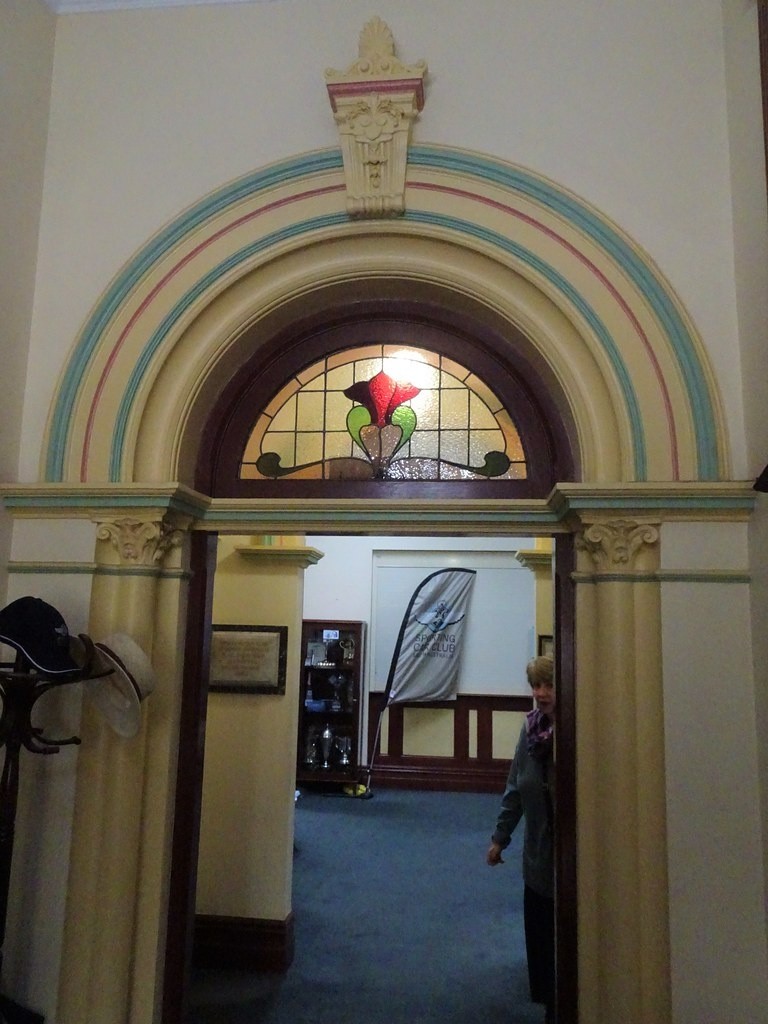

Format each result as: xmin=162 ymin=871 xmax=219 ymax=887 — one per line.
xmin=296 ymin=620 xmax=367 ymax=798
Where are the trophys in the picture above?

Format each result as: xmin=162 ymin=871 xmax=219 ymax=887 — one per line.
xmin=302 ymin=630 xmax=357 ymax=771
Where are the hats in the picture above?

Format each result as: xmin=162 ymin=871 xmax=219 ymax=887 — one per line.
xmin=1 ymin=597 xmax=91 ymax=675
xmin=79 ymin=632 xmax=153 ymax=737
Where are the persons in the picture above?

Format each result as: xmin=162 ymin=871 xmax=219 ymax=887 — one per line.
xmin=488 ymin=655 xmax=556 ymax=1004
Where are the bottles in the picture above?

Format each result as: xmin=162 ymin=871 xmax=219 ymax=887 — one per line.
xmin=304 ymin=672 xmax=314 ymax=707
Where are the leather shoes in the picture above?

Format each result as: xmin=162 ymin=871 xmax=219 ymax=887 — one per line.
xmin=544 ymin=1006 xmax=578 ymax=1024
